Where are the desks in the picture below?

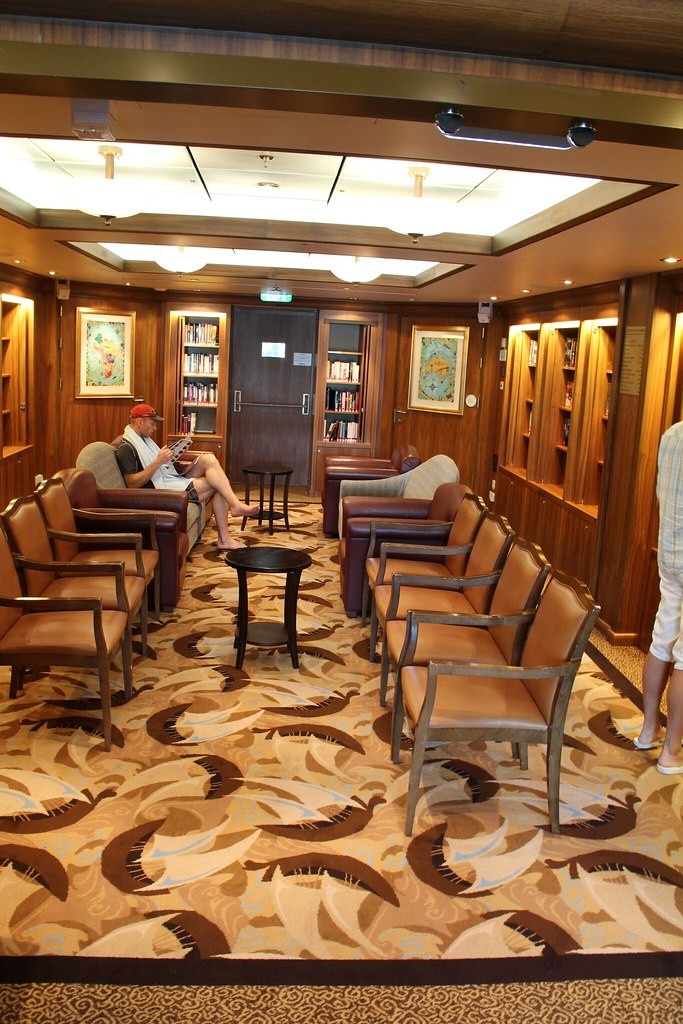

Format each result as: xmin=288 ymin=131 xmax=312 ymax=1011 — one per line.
xmin=241 ymin=464 xmax=294 ymax=535
xmin=224 ymin=546 xmax=312 ymax=669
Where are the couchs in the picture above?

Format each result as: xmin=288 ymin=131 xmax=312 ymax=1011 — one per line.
xmin=324 ymin=444 xmax=460 ymax=618
xmin=50 ymin=433 xmax=215 ymax=610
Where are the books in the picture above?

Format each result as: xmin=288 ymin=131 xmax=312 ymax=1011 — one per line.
xmin=185 ymin=353 xmax=219 ymax=374
xmin=327 ymin=387 xmax=358 ymax=412
xmin=529 ymin=340 xmax=537 ymax=364
xmin=186 ymin=323 xmax=217 ymax=345
xmin=327 ymin=361 xmax=359 ymax=383
xmin=563 ymin=418 xmax=570 ymax=446
xmin=528 ymin=411 xmax=532 ymax=431
xmin=165 ymin=434 xmax=193 ymax=467
xmin=170 ymin=454 xmax=203 ymax=476
xmin=565 ymin=382 xmax=573 ymax=407
xmin=182 ymin=413 xmax=214 ymax=435
xmin=606 ymin=383 xmax=610 ymax=415
xmin=565 ymin=337 xmax=577 ymax=367
xmin=324 ymin=420 xmax=358 ymax=441
xmin=184 ymin=382 xmax=218 ymax=404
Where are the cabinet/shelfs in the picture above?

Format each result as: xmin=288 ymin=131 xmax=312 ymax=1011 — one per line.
xmin=488 ymin=301 xmax=621 ymax=603
xmin=307 ymin=308 xmax=388 ymax=499
xmin=0 ymin=281 xmax=35 ymax=514
xmin=162 ymin=302 xmax=232 ymax=478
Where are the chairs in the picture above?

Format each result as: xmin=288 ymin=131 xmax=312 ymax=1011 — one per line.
xmin=0 ymin=477 xmax=162 ymax=753
xmin=361 ymin=493 xmax=602 ymax=836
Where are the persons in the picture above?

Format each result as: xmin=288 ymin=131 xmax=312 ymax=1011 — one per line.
xmin=634 ymin=421 xmax=683 ymax=774
xmin=115 ymin=405 xmax=260 ymax=550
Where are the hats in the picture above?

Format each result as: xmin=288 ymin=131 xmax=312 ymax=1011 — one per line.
xmin=130 ymin=404 xmax=165 ymax=421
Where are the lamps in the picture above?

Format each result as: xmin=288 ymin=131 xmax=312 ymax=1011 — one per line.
xmin=260 ymin=287 xmax=294 ymax=303
xmin=69 ymin=145 xmax=144 ymax=226
xmin=153 ymin=245 xmax=212 ymax=278
xmin=381 ymin=164 xmax=450 ymax=245
xmin=54 ymin=277 xmax=71 ymax=300
xmin=327 ymin=255 xmax=385 ymax=288
xmin=477 ymin=297 xmax=493 ymax=324
xmin=71 ymin=96 xmax=117 ymax=142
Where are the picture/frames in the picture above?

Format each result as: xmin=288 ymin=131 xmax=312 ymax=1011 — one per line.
xmin=74 ymin=306 xmax=136 ymax=400
xmin=407 ymin=324 xmax=470 ymax=416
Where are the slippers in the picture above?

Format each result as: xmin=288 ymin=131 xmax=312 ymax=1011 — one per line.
xmin=657 ymin=759 xmax=683 ymax=774
xmin=633 ymin=730 xmax=667 ymax=749
